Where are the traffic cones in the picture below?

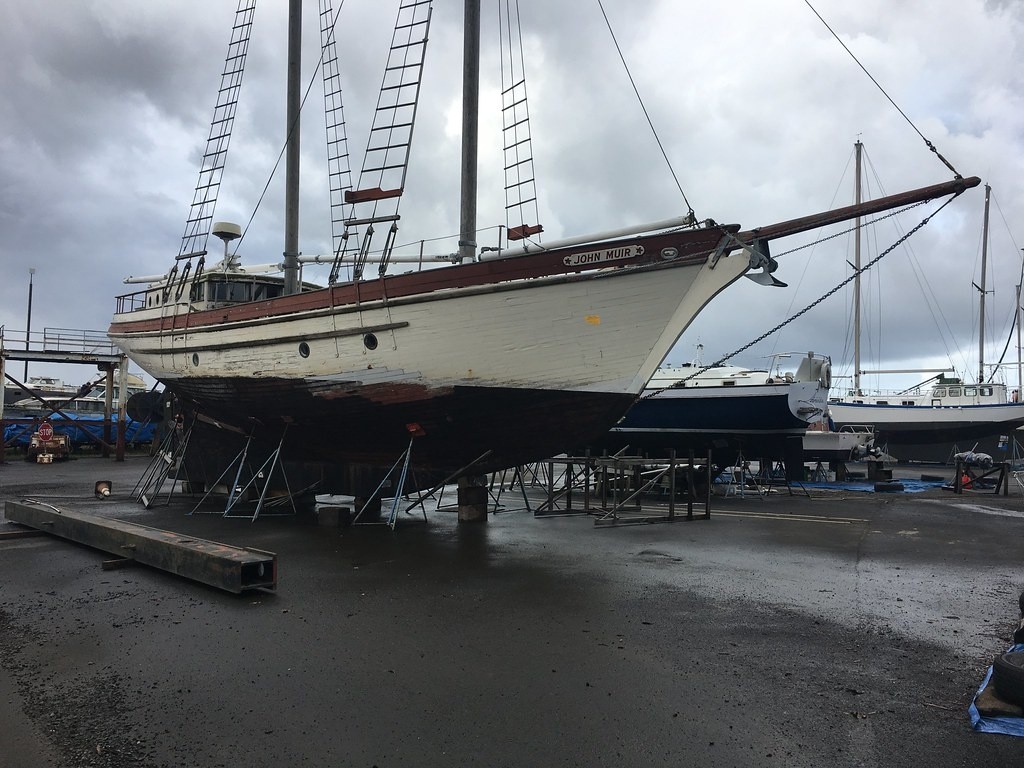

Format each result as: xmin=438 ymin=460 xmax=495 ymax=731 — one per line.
xmin=94 ymin=481 xmax=112 ymax=499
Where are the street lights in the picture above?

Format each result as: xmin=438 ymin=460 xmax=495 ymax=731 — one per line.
xmin=24 ymin=268 xmax=35 ymax=383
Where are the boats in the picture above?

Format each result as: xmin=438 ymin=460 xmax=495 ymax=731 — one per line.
xmin=12 ymin=383 xmax=149 ymax=413
xmin=565 ymin=340 xmax=875 ymax=463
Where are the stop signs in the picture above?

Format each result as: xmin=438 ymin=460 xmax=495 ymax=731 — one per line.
xmin=39 ymin=423 xmax=53 ymax=441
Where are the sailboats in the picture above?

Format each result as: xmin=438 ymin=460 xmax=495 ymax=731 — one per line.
xmin=105 ymin=0 xmax=975 ymax=497
xmin=767 ymin=142 xmax=1023 ymax=461
